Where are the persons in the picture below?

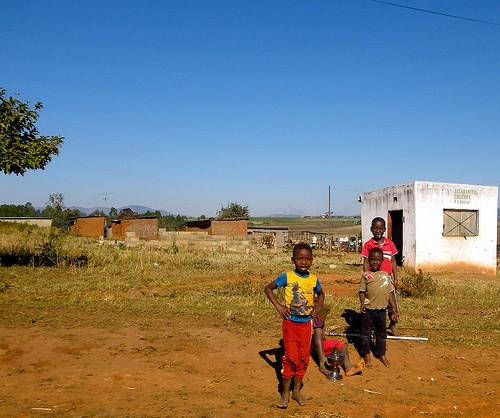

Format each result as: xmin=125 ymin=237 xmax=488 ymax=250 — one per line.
xmin=313 ymin=292 xmax=365 ymax=376
xmin=358 ymin=247 xmax=398 ymax=368
xmin=362 ymin=217 xmax=399 ymax=337
xmin=265 ymin=242 xmax=325 ymax=408
